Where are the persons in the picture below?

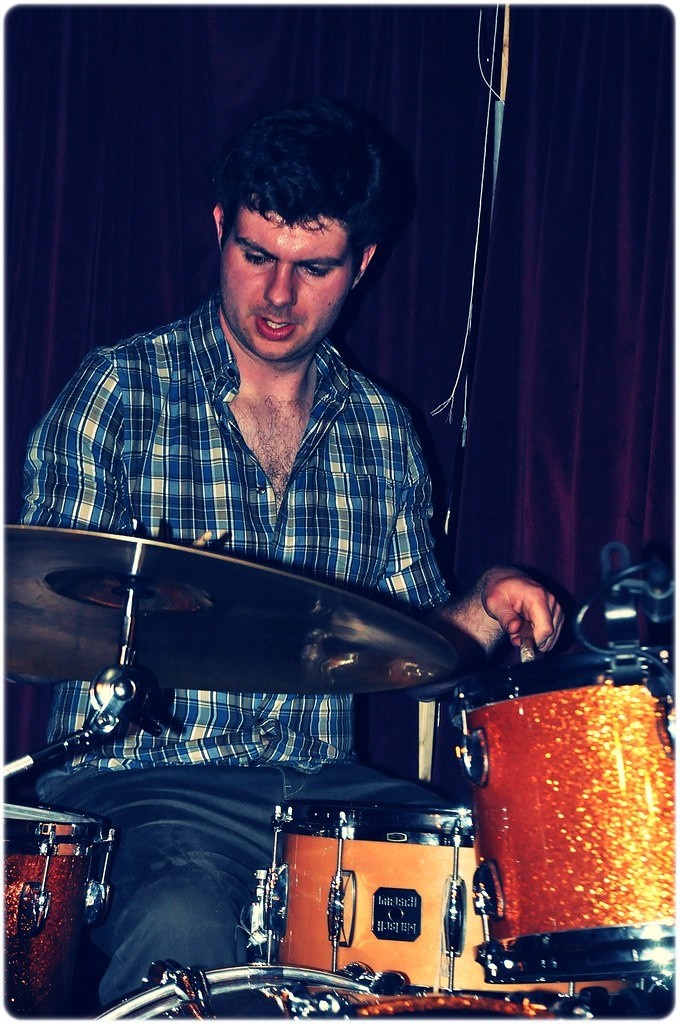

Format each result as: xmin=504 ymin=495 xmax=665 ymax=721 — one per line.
xmin=11 ymin=106 xmax=567 ymax=1011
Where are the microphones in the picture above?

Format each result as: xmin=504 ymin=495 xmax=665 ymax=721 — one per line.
xmin=641 ymin=560 xmax=673 ymax=623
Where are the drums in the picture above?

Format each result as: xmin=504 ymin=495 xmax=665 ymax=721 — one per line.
xmin=273 ymin=798 xmax=631 ymax=999
xmin=5 ymin=802 xmax=118 ymax=1020
xmin=92 ymin=958 xmax=592 ymax=1019
xmin=448 ymin=645 xmax=676 ymax=986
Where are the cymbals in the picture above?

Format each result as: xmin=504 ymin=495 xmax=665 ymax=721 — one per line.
xmin=5 ymin=526 xmax=459 ymax=693
